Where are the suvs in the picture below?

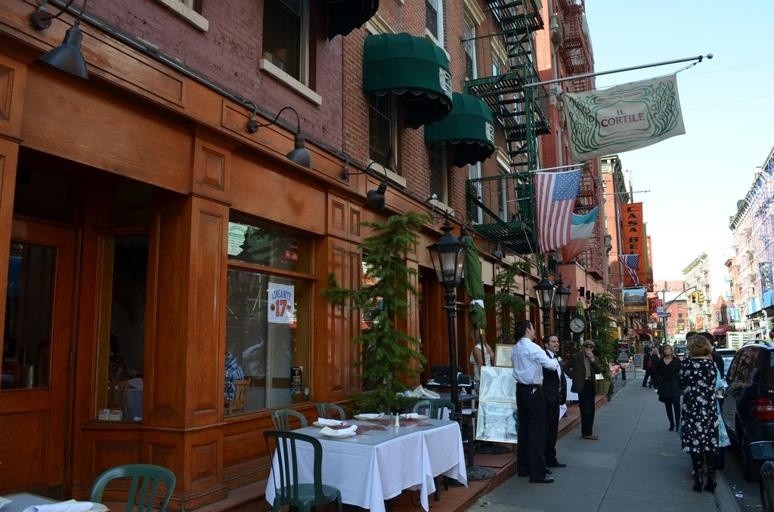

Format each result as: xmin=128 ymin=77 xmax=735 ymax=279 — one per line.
xmin=721 ymin=340 xmax=774 ymax=481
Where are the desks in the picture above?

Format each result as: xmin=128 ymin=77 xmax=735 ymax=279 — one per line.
xmin=397 ymin=392 xmax=477 ymax=418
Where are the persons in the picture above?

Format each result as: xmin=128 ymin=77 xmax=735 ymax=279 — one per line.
xmin=643 ymin=343 xmax=661 ymax=389
xmin=562 ymin=340 xmax=598 ymax=440
xmin=469 ymin=328 xmax=494 ymax=382
xmin=658 ymin=345 xmax=682 ymax=431
xmin=543 ymin=335 xmax=567 ymax=467
xmin=512 ymin=319 xmax=558 ymax=483
xmin=682 ymin=332 xmax=724 ymax=495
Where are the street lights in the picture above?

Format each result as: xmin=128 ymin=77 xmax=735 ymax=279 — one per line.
xmin=533 ymin=266 xmax=558 ymax=337
xmin=553 ymin=272 xmax=571 ymax=356
xmin=427 ymin=208 xmax=497 ymax=483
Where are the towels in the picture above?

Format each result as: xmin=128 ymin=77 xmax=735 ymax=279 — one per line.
xmin=319 ymin=425 xmax=358 ymax=436
xmin=22 ymin=501 xmax=98 ymax=511
xmin=400 ymin=413 xmax=428 ymax=419
xmin=356 ymin=413 xmax=384 ymax=419
xmin=313 ymin=418 xmax=347 ymax=426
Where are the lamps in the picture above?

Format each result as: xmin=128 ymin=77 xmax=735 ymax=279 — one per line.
xmin=245 ymin=107 xmax=314 ymax=169
xmin=474 ymin=234 xmax=503 ymax=259
xmin=427 ymin=210 xmax=469 ymax=242
xmin=344 ymin=161 xmax=386 ymax=207
xmin=28 ymin=0 xmax=91 ymax=80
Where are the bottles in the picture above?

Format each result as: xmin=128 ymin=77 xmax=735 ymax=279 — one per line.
xmin=389 ymin=411 xmax=394 ymax=427
xmin=394 ymin=412 xmax=400 ymax=427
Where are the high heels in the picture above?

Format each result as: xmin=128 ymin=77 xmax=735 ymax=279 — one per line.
xmin=705 ymin=474 xmax=718 ymax=495
xmin=691 ymin=473 xmax=704 ymax=493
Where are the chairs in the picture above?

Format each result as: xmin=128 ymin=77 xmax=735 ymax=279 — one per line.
xmin=273 ymin=408 xmax=308 ymax=430
xmin=224 ymin=377 xmax=252 ymax=415
xmin=317 ymin=404 xmax=345 ymax=421
xmin=107 ymin=379 xmax=129 ymax=416
xmin=264 ymin=431 xmax=342 ymax=512
xmin=413 ymin=399 xmax=455 ymax=490
xmin=91 ymin=465 xmax=177 ymax=511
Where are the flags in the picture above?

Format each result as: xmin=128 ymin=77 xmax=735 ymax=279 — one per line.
xmin=619 ymin=254 xmax=639 ymax=286
xmin=535 ymin=168 xmax=582 ymax=253
xmin=564 ymin=206 xmax=599 ymax=261
xmin=564 ymin=74 xmax=686 ymax=161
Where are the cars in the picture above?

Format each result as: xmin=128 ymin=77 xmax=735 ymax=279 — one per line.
xmin=716 ymin=348 xmax=739 ymax=378
xmin=684 ymin=351 xmax=690 ymax=361
xmin=673 ymin=346 xmax=689 ymax=361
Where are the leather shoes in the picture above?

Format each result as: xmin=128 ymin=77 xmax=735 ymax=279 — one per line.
xmin=668 ymin=423 xmax=674 ymax=432
xmin=528 ymin=474 xmax=555 ymax=483
xmin=675 ymin=424 xmax=680 ymax=431
xmin=546 ymin=461 xmax=567 ymax=468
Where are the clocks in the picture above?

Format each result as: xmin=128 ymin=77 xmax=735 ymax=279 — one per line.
xmin=569 ymin=318 xmax=586 ymax=352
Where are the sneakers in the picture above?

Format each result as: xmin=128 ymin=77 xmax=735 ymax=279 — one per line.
xmin=584 ymin=433 xmax=598 ymax=440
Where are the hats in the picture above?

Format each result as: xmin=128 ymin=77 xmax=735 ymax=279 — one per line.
xmin=582 ymin=339 xmax=595 ymax=347
